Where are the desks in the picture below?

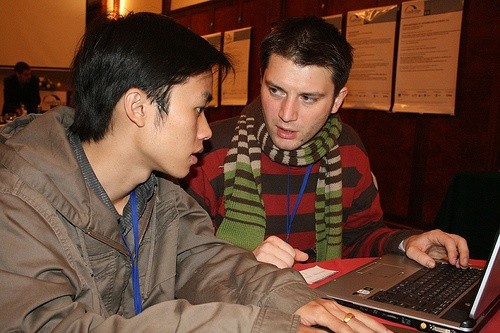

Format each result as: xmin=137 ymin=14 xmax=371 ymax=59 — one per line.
xmin=293 ymin=258 xmax=500 ymax=333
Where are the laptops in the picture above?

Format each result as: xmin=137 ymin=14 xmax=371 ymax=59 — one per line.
xmin=315 ymin=229 xmax=500 ymax=333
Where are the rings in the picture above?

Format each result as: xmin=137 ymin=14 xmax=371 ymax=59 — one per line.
xmin=343 ymin=312 xmax=356 ymax=322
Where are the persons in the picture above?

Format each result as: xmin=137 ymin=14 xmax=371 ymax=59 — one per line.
xmin=0 ymin=11 xmax=393 ymax=333
xmin=171 ymin=14 xmax=471 ymax=271
xmin=0 ymin=61 xmax=41 ymax=119
